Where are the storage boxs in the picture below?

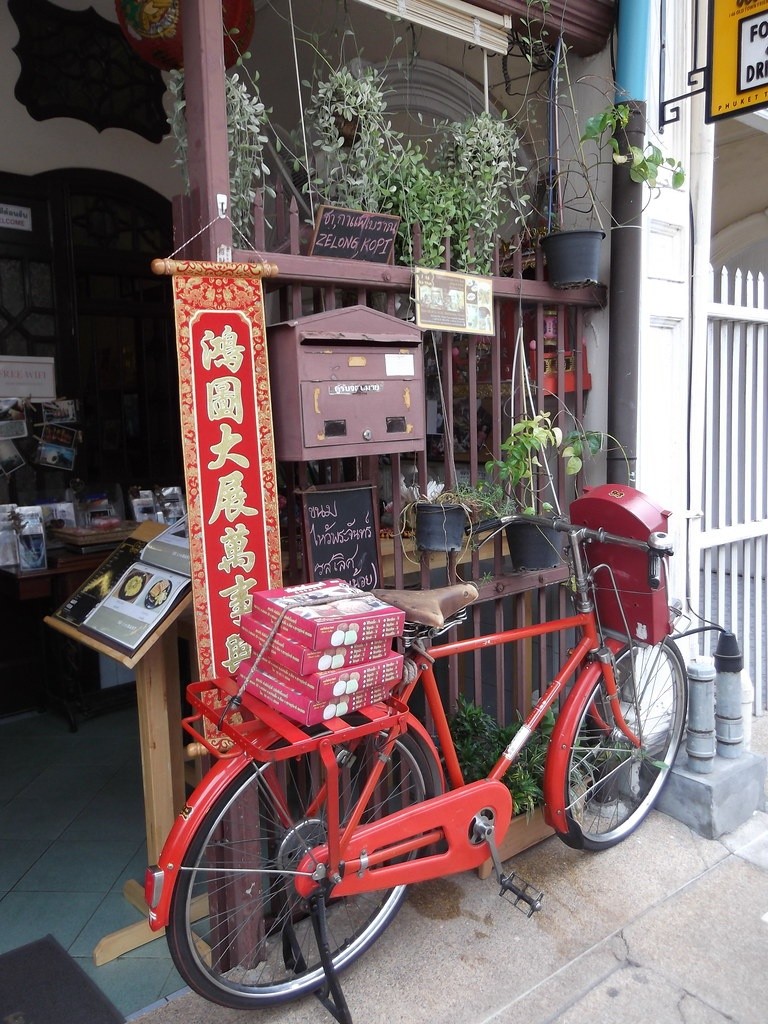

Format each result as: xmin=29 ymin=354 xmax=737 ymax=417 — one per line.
xmin=251 ymin=646 xmax=404 ymax=703
xmin=237 ymin=658 xmax=389 ymax=727
xmin=251 ymin=578 xmax=404 ymax=651
xmin=240 ymin=613 xmax=394 ymax=676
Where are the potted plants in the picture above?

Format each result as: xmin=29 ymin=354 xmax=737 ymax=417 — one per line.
xmin=432 ymin=694 xmax=594 ymax=880
xmin=388 ymin=491 xmax=503 ymax=584
xmin=484 ymin=411 xmax=603 ymax=571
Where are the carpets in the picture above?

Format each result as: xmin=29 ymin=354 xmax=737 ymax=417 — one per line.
xmin=0 ymin=934 xmax=127 ymax=1024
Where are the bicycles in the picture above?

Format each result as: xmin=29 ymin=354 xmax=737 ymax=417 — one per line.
xmin=144 ymin=481 xmax=692 ymax=1024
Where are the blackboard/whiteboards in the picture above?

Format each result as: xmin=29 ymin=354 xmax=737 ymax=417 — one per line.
xmin=300 ymin=483 xmax=385 ymax=590
xmin=309 ymin=205 xmax=401 ymax=265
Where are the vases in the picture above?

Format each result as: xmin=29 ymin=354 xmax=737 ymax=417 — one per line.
xmin=539 ymin=229 xmax=606 ymax=289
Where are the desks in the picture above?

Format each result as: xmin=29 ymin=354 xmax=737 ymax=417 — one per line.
xmin=95 ymin=524 xmax=515 ymax=968
xmin=0 ymin=544 xmax=188 ymax=734
